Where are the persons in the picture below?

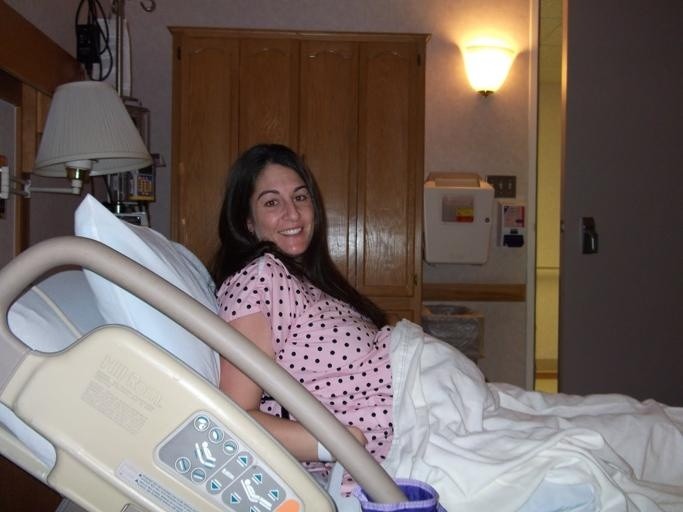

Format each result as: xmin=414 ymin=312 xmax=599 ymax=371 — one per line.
xmin=211 ymin=143 xmax=489 ymax=495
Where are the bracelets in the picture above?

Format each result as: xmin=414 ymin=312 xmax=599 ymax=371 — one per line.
xmin=318 ymin=441 xmax=332 ymax=461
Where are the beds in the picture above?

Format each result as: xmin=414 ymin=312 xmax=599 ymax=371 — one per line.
xmin=0 ymin=235 xmax=409 ymax=512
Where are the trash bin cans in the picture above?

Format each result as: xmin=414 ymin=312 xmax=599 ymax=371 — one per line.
xmin=422 ymin=303 xmax=485 ymax=367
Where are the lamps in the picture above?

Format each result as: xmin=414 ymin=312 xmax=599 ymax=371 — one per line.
xmin=453 ymin=23 xmax=527 ymax=100
xmin=1 ymin=81 xmax=154 ymax=201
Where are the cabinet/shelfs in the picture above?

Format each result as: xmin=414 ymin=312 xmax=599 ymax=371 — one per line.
xmin=165 ymin=23 xmax=433 ymax=331
xmin=0 ymin=0 xmax=86 ymax=270
